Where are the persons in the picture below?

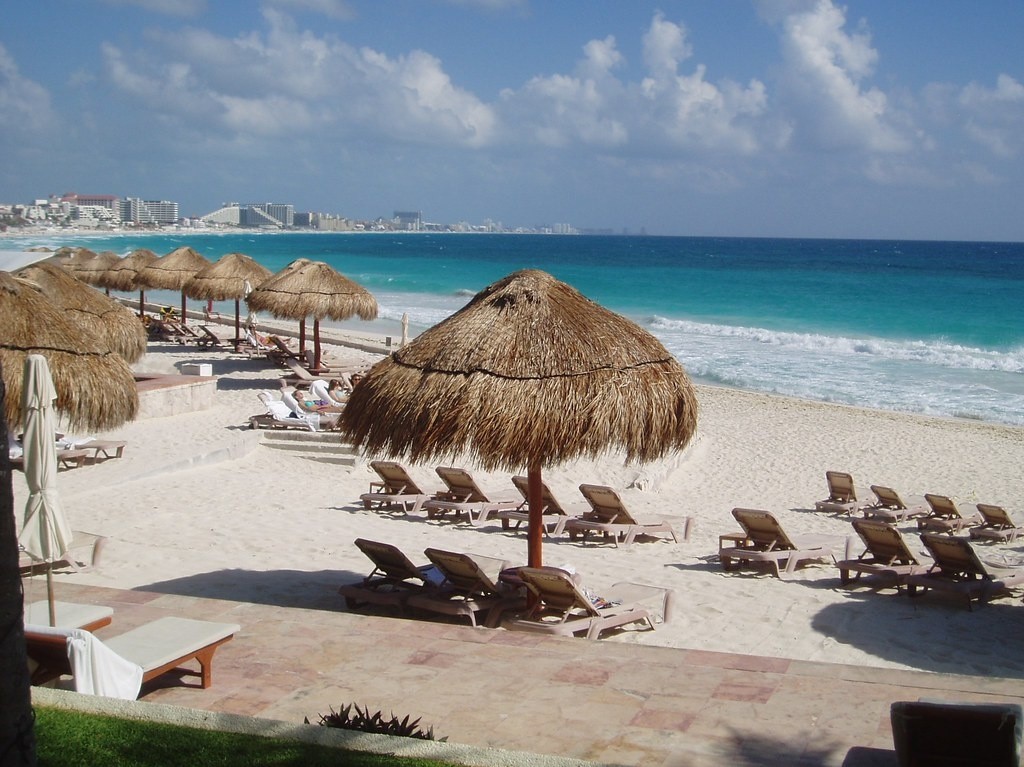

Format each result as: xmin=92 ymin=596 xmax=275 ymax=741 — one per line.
xmin=143 ymin=314 xmax=180 ymax=329
xmin=248 ymin=325 xmax=291 ymax=346
xmin=292 ymin=373 xmax=364 ymax=415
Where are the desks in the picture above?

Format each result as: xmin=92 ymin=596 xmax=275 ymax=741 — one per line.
xmin=719 ymin=532 xmax=751 ymax=564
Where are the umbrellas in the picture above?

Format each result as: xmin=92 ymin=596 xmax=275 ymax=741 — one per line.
xmin=17 ymin=353 xmax=74 ymax=627
xmin=400 ymin=312 xmax=409 ymax=347
xmin=242 ymin=280 xmax=260 ymax=357
xmin=340 ymin=269 xmax=698 ymax=622
xmin=1 ymin=247 xmax=379 ymax=434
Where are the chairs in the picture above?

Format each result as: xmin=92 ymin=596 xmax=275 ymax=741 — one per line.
xmin=19 ymin=530 xmax=109 ymax=572
xmin=23 ymin=601 xmax=117 ymax=685
xmin=890 ymin=700 xmax=1023 ymax=767
xmin=23 ymin=616 xmax=241 ymax=702
xmin=862 ymin=485 xmax=929 ymax=525
xmin=915 ymin=494 xmax=982 ymax=536
xmin=7 ymin=431 xmax=128 ymax=471
xmin=136 ymin=312 xmax=373 ymax=430
xmin=838 ymin=519 xmax=921 ymax=585
xmin=968 ymin=504 xmax=1024 ymax=545
xmin=719 ymin=508 xmax=839 ymax=578
xmin=340 ymin=460 xmax=694 ymax=642
xmin=905 ymin=534 xmax=1005 ymax=611
xmin=814 ymin=471 xmax=870 ymax=518
